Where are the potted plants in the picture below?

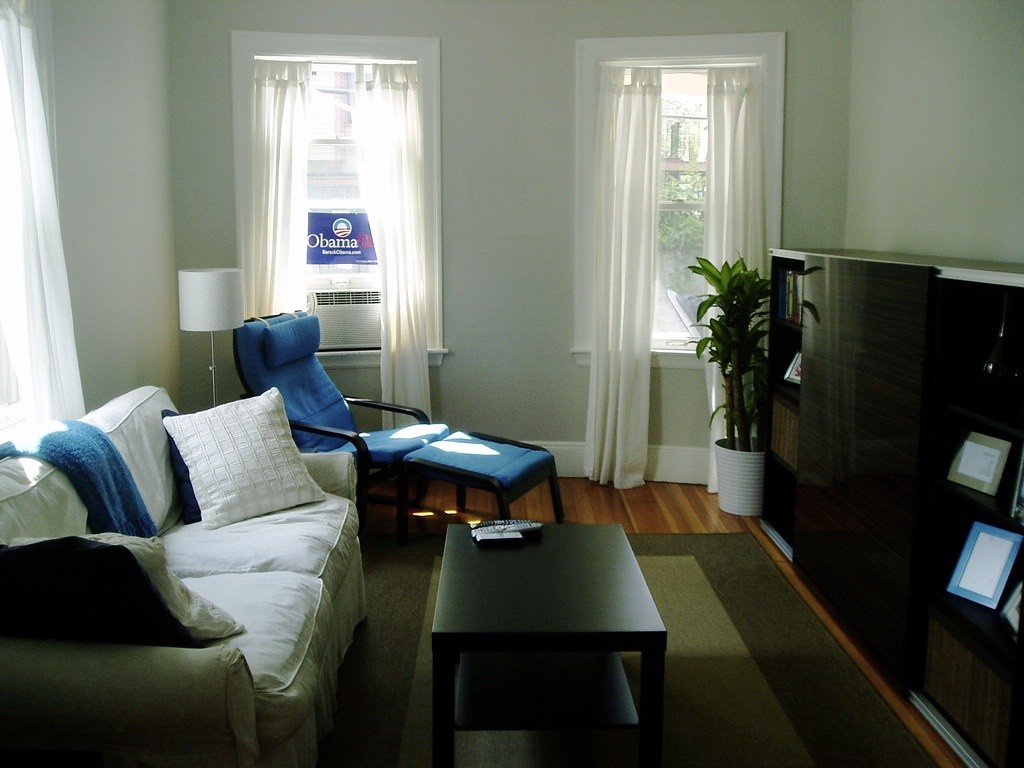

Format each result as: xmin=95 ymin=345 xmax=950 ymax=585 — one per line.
xmin=686 ymin=258 xmax=826 ymax=514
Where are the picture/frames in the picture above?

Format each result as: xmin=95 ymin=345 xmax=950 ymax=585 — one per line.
xmin=947 ymin=521 xmax=1024 ymax=609
xmin=948 ymin=432 xmax=1012 ymax=495
xmin=1011 ymin=448 xmax=1024 ymax=518
xmin=1002 ymin=582 xmax=1023 ymax=633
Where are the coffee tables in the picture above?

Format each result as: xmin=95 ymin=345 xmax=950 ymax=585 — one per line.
xmin=434 ymin=523 xmax=667 ymax=768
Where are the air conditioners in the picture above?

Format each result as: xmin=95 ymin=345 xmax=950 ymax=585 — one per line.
xmin=307 ymin=290 xmax=382 ymax=350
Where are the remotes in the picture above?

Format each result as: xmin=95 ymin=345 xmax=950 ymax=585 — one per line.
xmin=470 ymin=518 xmax=544 ymax=544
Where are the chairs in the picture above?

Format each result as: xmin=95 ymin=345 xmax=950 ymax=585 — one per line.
xmin=231 ymin=311 xmax=448 ymax=539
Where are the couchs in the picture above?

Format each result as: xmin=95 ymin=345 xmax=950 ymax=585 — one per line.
xmin=0 ymin=386 xmax=365 ymax=768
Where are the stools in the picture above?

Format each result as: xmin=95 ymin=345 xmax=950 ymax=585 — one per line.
xmin=404 ymin=430 xmax=566 ymax=523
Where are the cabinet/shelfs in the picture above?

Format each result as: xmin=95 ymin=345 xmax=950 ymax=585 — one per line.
xmin=764 ymin=247 xmax=1024 ymax=768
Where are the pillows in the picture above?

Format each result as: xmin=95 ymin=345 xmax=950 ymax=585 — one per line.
xmin=12 ymin=533 xmax=245 ymax=639
xmin=163 ymin=387 xmax=326 ymax=522
xmin=161 ymin=409 xmax=201 ymax=523
xmin=0 ymin=537 xmax=191 ymax=648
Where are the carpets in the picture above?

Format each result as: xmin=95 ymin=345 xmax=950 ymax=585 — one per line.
xmin=313 ymin=533 xmax=939 ymax=767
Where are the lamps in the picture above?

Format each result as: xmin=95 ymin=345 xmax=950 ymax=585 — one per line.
xmin=179 ymin=268 xmax=243 ymax=406
